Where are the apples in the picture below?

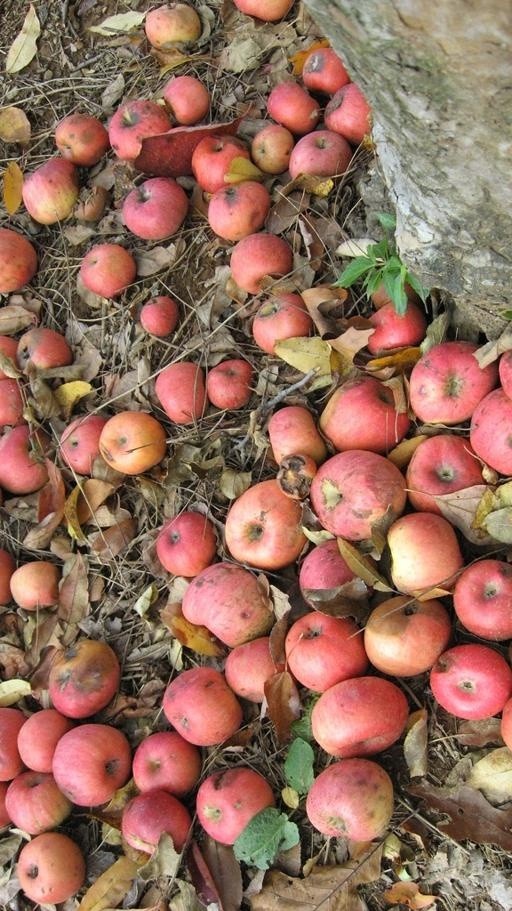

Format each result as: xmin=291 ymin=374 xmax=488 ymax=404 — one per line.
xmin=0 ymin=47 xmax=512 ymax=906
xmin=233 ymin=0 xmax=294 ymax=22
xmin=144 ymin=2 xmax=203 ymax=51
